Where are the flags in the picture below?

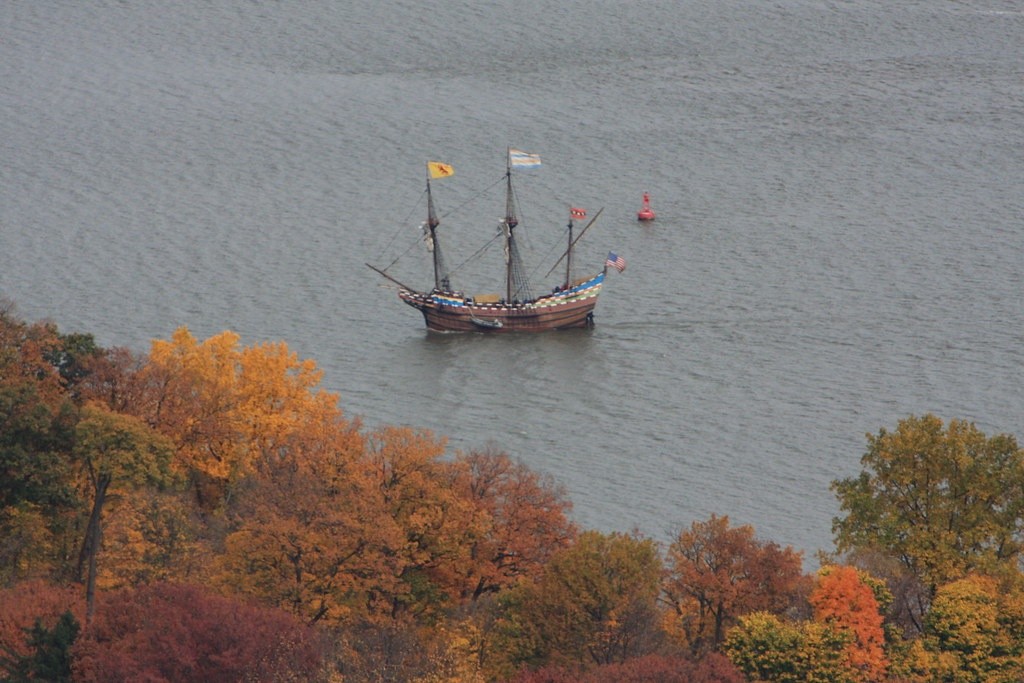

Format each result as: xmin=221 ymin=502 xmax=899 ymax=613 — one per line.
xmin=510 ymin=147 xmax=544 ymax=169
xmin=606 ymin=252 xmax=626 ymax=273
xmin=428 ymin=162 xmax=455 ymax=178
xmin=570 ymin=208 xmax=586 ymax=218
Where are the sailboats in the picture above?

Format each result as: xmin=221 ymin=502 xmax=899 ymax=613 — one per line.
xmin=363 ymin=145 xmax=625 ymax=337
